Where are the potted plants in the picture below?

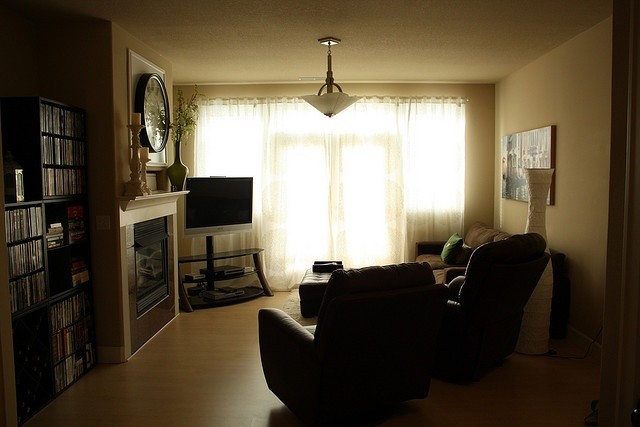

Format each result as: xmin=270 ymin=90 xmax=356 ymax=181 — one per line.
xmin=165 ymin=82 xmax=201 ymax=190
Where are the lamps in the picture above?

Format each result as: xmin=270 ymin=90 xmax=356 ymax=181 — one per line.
xmin=298 ymin=34 xmax=364 ymax=118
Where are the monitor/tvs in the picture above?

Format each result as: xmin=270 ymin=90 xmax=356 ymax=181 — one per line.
xmin=184 ymin=175 xmax=252 ymax=236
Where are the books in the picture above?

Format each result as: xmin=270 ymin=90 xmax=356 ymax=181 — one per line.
xmin=52 ymin=315 xmax=91 ymax=363
xmin=51 ymin=291 xmax=86 ymax=332
xmin=45 ymin=223 xmax=64 ymax=249
xmin=42 ymin=135 xmax=84 ymax=166
xmin=40 ymin=102 xmax=84 ymax=138
xmin=55 ymin=342 xmax=93 ymax=393
xmin=8 ymin=240 xmax=43 ymax=279
xmin=71 ymin=256 xmax=89 ymax=287
xmin=5 ymin=207 xmax=42 ymax=243
xmin=9 ymin=271 xmax=47 ymax=313
xmin=42 ymin=167 xmax=82 ymax=196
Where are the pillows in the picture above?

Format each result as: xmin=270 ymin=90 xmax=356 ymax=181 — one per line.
xmin=441 ymin=233 xmax=463 ymax=262
xmin=458 ymin=243 xmax=471 ymax=260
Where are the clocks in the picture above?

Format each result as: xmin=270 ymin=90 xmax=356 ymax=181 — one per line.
xmin=137 ymin=72 xmax=170 ymax=153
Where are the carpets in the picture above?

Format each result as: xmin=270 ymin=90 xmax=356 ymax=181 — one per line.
xmin=281 ymin=288 xmax=317 ymax=327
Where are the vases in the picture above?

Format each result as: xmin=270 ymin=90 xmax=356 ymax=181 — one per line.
xmin=519 ymin=166 xmax=556 ymax=354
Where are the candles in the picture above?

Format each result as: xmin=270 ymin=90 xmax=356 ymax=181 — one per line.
xmin=139 ymin=146 xmax=149 ymax=156
xmin=131 ymin=112 xmax=141 ymax=122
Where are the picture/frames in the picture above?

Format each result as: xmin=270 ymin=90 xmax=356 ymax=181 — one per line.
xmin=501 ymin=126 xmax=556 ymax=207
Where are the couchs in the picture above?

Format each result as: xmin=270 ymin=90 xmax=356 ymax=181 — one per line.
xmin=433 ymin=233 xmax=551 ymax=385
xmin=258 ymin=263 xmax=441 ymax=423
xmin=413 ymin=221 xmax=510 ymax=284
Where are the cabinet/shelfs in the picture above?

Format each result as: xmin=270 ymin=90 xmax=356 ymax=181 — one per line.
xmin=36 ymin=96 xmax=76 ymax=198
xmin=178 ymin=248 xmax=274 ymax=311
xmin=53 ymin=287 xmax=101 ymax=392
xmin=6 ymin=203 xmax=49 ymax=317
xmin=43 ymin=200 xmax=88 ymax=297
